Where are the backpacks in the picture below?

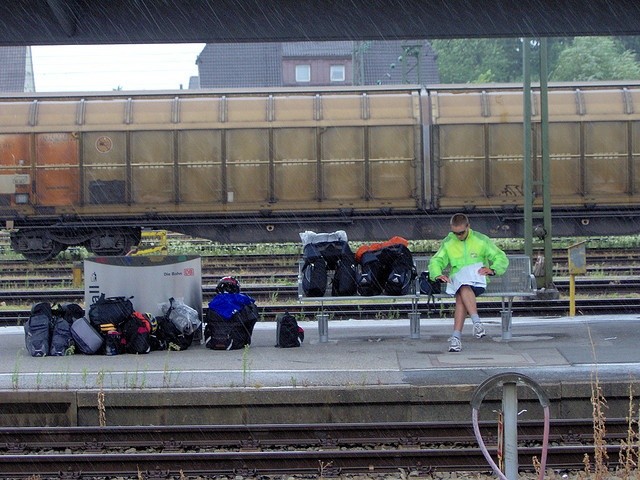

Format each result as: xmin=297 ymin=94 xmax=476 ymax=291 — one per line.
xmin=385 ymin=245 xmax=417 ymax=296
xmin=202 ymin=304 xmax=260 ymax=350
xmin=420 ymin=272 xmax=441 ymax=294
xmin=124 ymin=312 xmax=151 ymax=354
xmin=359 ymin=251 xmax=384 ymax=295
xmin=89 ymin=293 xmax=135 ymax=331
xmin=52 ymin=303 xmax=85 ymax=356
xmin=24 ymin=301 xmax=52 ymax=357
xmin=301 ymin=243 xmax=327 ymax=297
xmin=329 ymin=243 xmax=358 ymax=295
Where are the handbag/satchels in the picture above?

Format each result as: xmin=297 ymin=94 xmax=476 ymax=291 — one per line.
xmin=275 ymin=309 xmax=304 ymax=347
xmin=70 ymin=316 xmax=104 ymax=355
xmin=156 ymin=298 xmax=201 ymax=352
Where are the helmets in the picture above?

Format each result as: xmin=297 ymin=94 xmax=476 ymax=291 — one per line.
xmin=216 ymin=277 xmax=241 ymax=294
xmin=141 ymin=312 xmax=157 ymax=335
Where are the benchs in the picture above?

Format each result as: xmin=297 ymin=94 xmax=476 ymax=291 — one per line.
xmin=298 ymin=254 xmax=537 ymax=342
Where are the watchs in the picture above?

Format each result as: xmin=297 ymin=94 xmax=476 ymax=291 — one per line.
xmin=491 ymin=269 xmax=496 ymax=277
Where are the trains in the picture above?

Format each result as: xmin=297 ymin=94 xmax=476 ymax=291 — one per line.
xmin=0 ymin=81 xmax=640 ymax=262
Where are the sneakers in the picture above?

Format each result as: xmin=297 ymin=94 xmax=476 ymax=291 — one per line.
xmin=447 ymin=336 xmax=462 ymax=352
xmin=473 ymin=321 xmax=486 ymax=339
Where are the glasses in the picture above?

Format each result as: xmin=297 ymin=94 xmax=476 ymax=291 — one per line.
xmin=450 ymin=223 xmax=468 ymax=235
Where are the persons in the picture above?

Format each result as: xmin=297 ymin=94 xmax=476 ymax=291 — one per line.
xmin=428 ymin=213 xmax=509 ymax=351
